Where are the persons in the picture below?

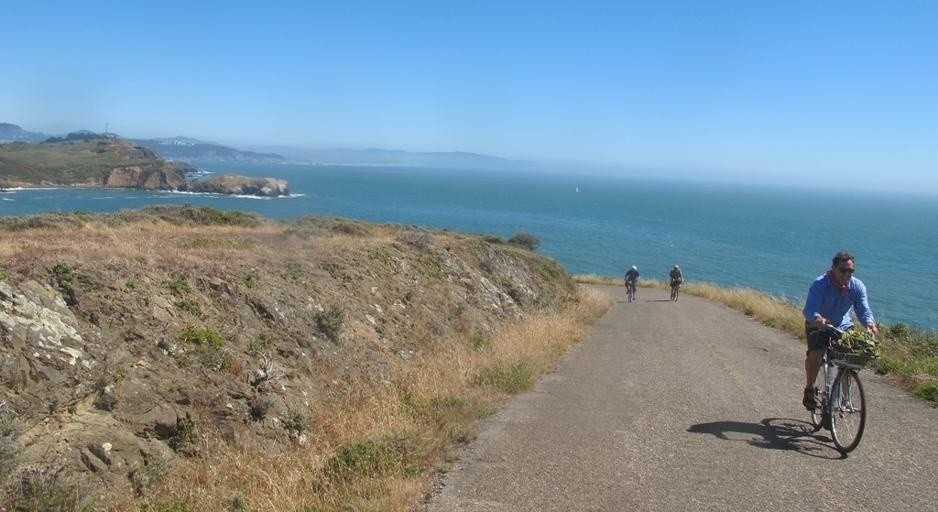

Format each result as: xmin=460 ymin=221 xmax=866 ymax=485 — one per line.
xmin=669 ymin=264 xmax=683 ymax=297
xmin=800 ymin=252 xmax=881 ymax=411
xmin=623 ymin=263 xmax=640 ymax=301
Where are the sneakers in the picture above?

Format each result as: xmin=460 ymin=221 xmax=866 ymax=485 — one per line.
xmin=841 ymin=400 xmax=861 ymax=412
xmin=803 ymin=388 xmax=817 ymax=409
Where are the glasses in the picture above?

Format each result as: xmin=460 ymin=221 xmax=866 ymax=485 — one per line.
xmin=839 ymin=268 xmax=854 ymax=273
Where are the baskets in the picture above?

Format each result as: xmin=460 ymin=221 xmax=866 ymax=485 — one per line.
xmin=828 ymin=347 xmax=870 ymax=370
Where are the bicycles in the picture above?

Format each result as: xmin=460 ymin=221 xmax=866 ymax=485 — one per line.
xmin=670 ymin=280 xmax=682 ymax=301
xmin=625 ymin=278 xmax=638 ymax=302
xmin=809 ymin=321 xmax=876 ymax=454
